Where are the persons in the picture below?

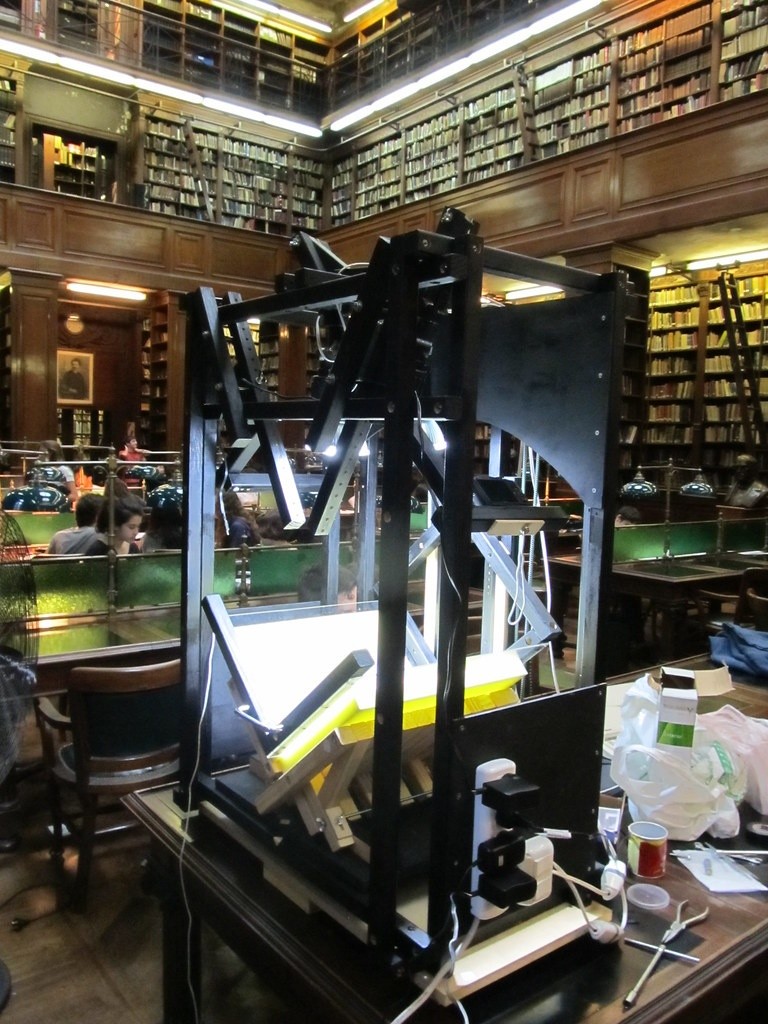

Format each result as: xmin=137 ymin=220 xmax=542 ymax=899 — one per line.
xmin=290 ymin=492 xmax=323 ymax=544
xmin=374 ymin=466 xmax=421 ymax=513
xmin=343 ymin=561 xmax=376 ymax=612
xmin=69 ymin=462 xmax=106 ymax=507
xmin=413 ymin=483 xmax=428 ymax=502
xmin=295 ymin=564 xmax=353 ymax=617
xmin=140 ymin=496 xmax=187 ymax=553
xmin=250 ymin=508 xmax=297 ymax=549
xmin=32 ymin=440 xmax=78 ymax=507
xmin=215 ymin=490 xmax=259 ymax=549
xmin=85 ymin=498 xmax=141 ymax=557
xmin=102 ymin=477 xmax=144 ymax=515
xmin=613 ymin=506 xmax=641 ymax=525
xmin=44 ymin=493 xmax=109 ymax=557
xmin=118 ymin=437 xmax=147 ymax=461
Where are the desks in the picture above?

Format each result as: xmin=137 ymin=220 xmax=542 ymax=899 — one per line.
xmin=2 ymin=565 xmax=540 ymax=811
xmin=123 ymin=648 xmax=767 ymax=1024
xmin=539 ymin=529 xmax=767 ymax=659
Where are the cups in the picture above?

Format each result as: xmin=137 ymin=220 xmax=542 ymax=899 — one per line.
xmin=626 ymin=820 xmax=669 ymax=879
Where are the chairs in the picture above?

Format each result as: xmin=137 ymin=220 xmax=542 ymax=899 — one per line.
xmin=694 ymin=566 xmax=767 ymax=647
xmin=34 ymin=657 xmax=186 ymax=904
xmin=745 ymin=588 xmax=768 ymax=634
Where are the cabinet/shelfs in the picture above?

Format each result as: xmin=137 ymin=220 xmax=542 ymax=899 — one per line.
xmin=1 ymin=0 xmax=767 ymax=504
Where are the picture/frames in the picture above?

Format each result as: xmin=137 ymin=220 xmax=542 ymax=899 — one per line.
xmin=55 ymin=348 xmax=95 ymax=409
